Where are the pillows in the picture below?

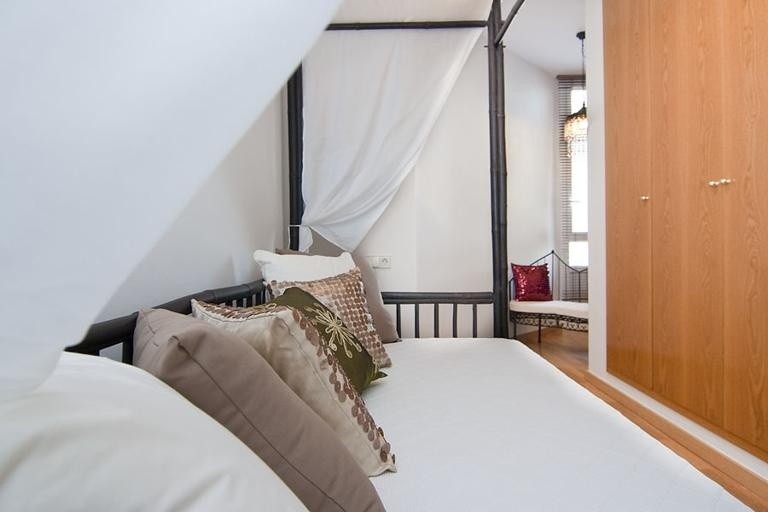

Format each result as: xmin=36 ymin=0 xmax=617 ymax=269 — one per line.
xmin=510 ymin=262 xmax=554 ymax=302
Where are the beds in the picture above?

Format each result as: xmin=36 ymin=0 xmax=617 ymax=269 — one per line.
xmin=509 ymin=250 xmax=590 ymax=344
xmin=0 ymin=281 xmax=756 ymax=510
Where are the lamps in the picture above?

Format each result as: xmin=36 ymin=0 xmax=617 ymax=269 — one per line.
xmin=564 ymin=30 xmax=588 ymax=158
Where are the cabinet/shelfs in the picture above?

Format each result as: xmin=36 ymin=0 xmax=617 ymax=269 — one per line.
xmin=604 ymin=2 xmax=766 ymax=458
